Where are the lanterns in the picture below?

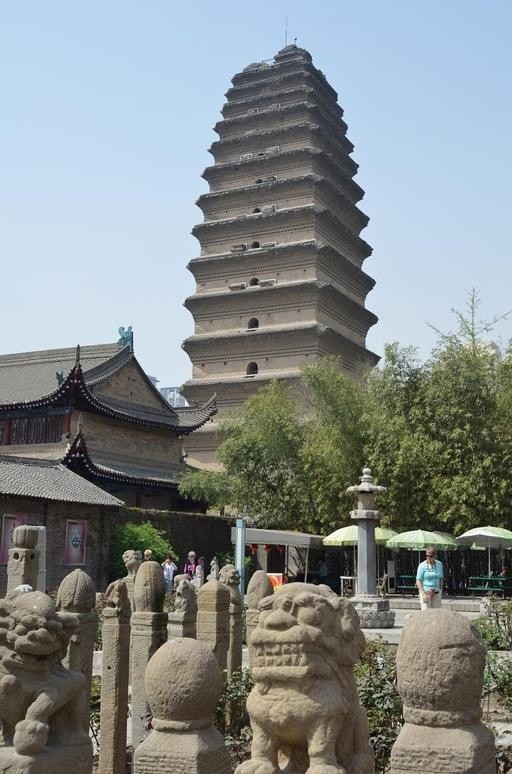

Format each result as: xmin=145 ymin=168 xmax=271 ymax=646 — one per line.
xmin=262 ymin=544 xmax=271 ymax=554
xmin=276 ymin=545 xmax=284 ymax=553
xmin=250 ymin=544 xmax=258 ymax=554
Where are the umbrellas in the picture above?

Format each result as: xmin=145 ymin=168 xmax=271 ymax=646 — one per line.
xmin=430 ymin=530 xmax=467 ymax=556
xmin=385 ymin=529 xmax=457 ymax=568
xmin=454 ymin=524 xmax=511 ymax=578
xmin=321 ymin=525 xmax=396 ymax=572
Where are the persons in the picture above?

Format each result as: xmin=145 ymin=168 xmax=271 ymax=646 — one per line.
xmin=161 ymin=555 xmax=177 ymax=593
xmin=415 ymin=546 xmax=444 ymax=610
xmin=184 ymin=550 xmax=199 ymax=580
xmin=143 ymin=549 xmax=152 ymax=562
xmin=197 ymin=556 xmax=211 ymax=574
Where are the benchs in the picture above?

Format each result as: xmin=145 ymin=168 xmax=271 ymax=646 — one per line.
xmin=467 ymin=586 xmax=510 ymax=591
xmin=398 ymin=584 xmax=418 ymax=588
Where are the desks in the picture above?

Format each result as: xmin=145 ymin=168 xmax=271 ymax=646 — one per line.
xmin=469 ymin=576 xmax=507 ymax=601
xmin=400 ymin=575 xmax=417 ymax=599
xmin=340 ymin=576 xmax=358 ymax=597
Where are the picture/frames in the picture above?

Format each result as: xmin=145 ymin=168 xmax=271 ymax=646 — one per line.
xmin=63 ymin=519 xmax=87 ymax=567
xmin=0 ymin=514 xmax=27 ymax=566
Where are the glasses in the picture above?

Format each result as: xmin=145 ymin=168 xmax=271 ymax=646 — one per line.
xmin=426 ymin=555 xmax=433 ymax=559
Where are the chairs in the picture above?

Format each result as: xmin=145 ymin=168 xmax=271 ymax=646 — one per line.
xmin=376 ymin=574 xmax=389 ymax=599
